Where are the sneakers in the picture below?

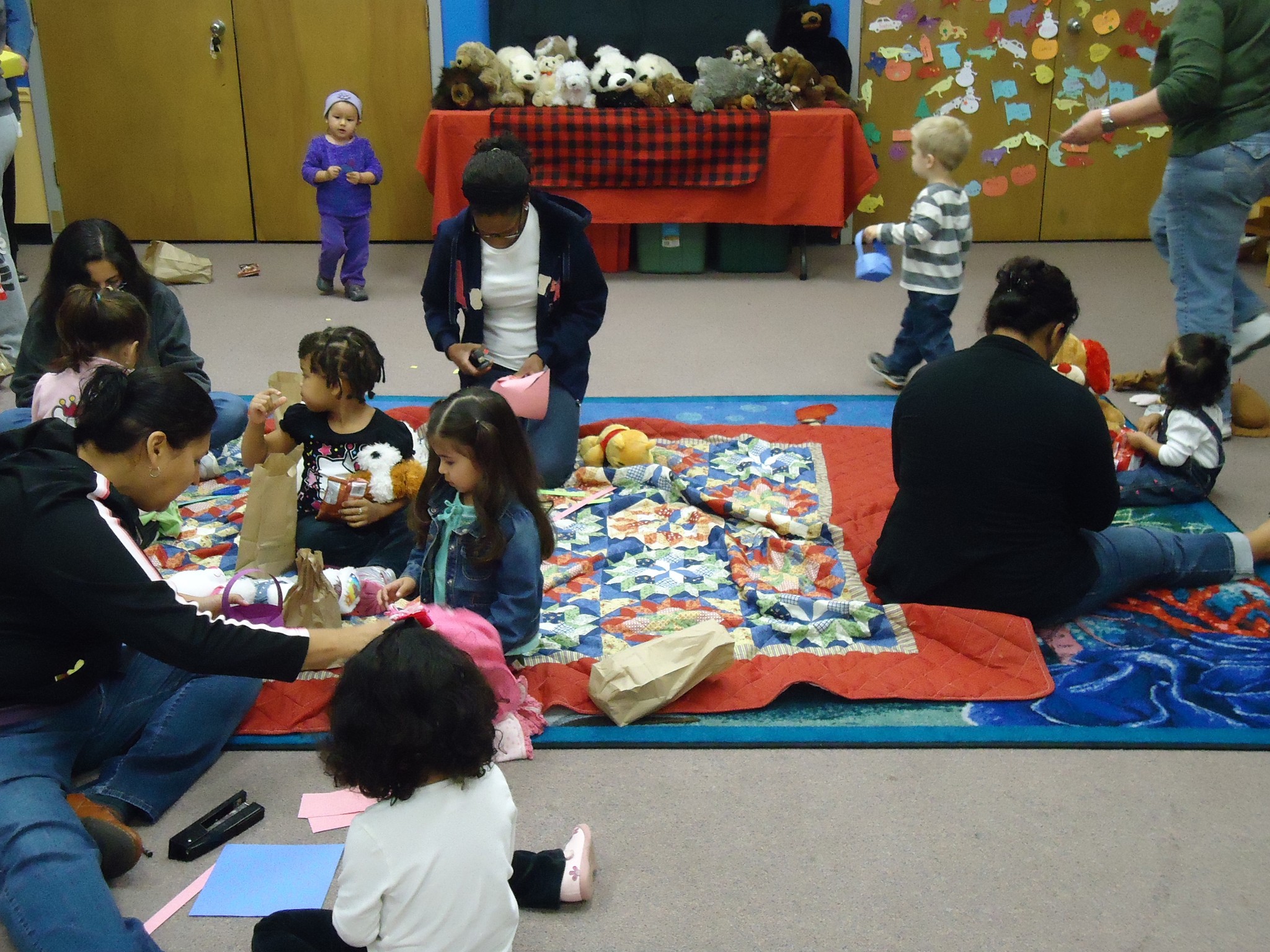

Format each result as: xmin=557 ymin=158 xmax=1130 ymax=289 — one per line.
xmin=317 ymin=274 xmax=334 ymax=292
xmin=868 ymin=352 xmax=906 ymax=389
xmin=345 ymin=285 xmax=368 ymax=301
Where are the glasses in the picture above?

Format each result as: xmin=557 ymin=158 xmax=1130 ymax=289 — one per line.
xmin=471 ymin=203 xmax=523 ymax=239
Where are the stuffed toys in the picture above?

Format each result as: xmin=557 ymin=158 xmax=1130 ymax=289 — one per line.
xmin=431 ymin=3 xmax=860 ymax=112
xmin=1050 ymin=334 xmax=1126 ymax=432
xmin=580 ymin=424 xmax=656 ymax=469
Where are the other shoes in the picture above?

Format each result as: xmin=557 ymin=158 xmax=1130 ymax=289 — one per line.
xmin=559 ymin=823 xmax=593 ymax=903
xmin=64 ymin=794 xmax=143 ymax=881
xmin=17 ymin=270 xmax=28 ymax=282
xmin=1228 ymin=312 xmax=1270 ymax=365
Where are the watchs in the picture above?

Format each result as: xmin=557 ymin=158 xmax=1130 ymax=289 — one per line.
xmin=1100 ymin=107 xmax=1117 ymax=134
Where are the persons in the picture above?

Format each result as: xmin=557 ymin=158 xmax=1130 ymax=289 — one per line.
xmin=866 ymin=253 xmax=1270 ymax=626
xmin=0 ymin=0 xmax=608 ymax=952
xmin=1046 ymin=0 xmax=1270 ymax=364
xmin=1115 ymin=332 xmax=1231 ymax=504
xmin=862 ymin=115 xmax=974 ymax=389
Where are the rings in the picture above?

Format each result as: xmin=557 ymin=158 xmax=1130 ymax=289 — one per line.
xmin=358 ymin=507 xmax=362 ymax=515
